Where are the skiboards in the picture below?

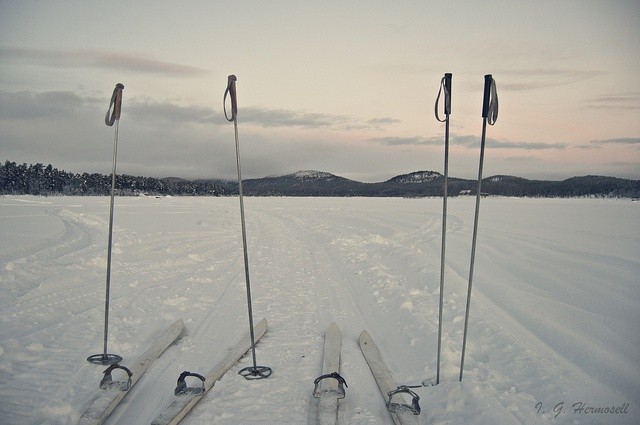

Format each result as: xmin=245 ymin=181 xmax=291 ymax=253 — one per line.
xmin=312 ymin=321 xmax=421 ymax=425
xmin=77 ymin=317 xmax=268 ymax=424
